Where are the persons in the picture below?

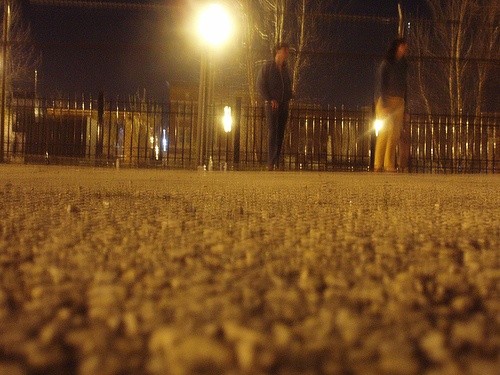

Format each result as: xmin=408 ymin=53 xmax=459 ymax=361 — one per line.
xmin=375 ymin=38 xmax=409 ymax=173
xmin=256 ymin=42 xmax=292 ymax=171
